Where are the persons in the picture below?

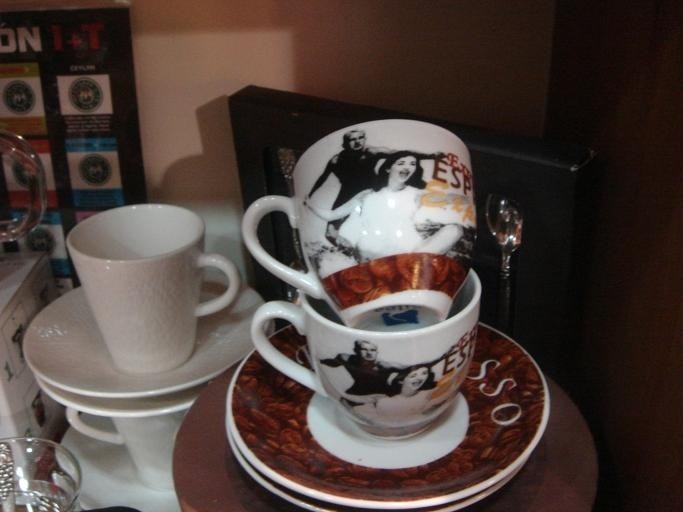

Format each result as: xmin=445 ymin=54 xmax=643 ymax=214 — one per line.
xmin=319 ymin=339 xmax=459 ymax=409
xmin=300 ymin=128 xmax=447 ymax=250
xmin=348 ymin=349 xmax=475 ymax=426
xmin=300 ymin=147 xmax=471 ymax=262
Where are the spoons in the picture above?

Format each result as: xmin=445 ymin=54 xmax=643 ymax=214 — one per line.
xmin=485 ymin=187 xmax=532 ymax=326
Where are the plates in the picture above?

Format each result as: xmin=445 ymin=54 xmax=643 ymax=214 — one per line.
xmin=224 ymin=314 xmax=553 ymax=512
xmin=16 ymin=278 xmax=276 ymax=422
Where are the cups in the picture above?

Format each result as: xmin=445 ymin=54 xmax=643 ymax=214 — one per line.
xmin=249 ymin=268 xmax=493 ymax=435
xmin=245 ymin=121 xmax=475 ymax=319
xmin=63 ymin=408 xmax=209 ymax=493
xmin=62 ymin=203 xmax=239 ymax=371
